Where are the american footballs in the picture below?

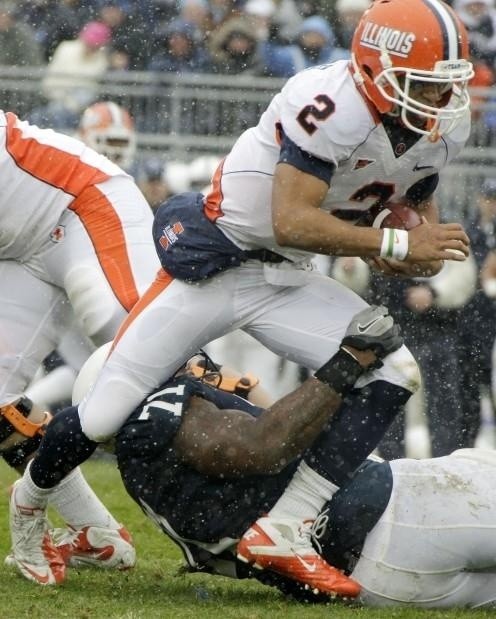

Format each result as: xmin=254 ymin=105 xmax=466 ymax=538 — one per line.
xmin=358 ymin=204 xmax=421 ymax=262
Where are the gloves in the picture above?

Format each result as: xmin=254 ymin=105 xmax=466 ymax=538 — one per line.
xmin=343 ymin=305 xmax=405 ymax=370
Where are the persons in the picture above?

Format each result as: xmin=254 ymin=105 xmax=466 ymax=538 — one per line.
xmin=0 ymin=1 xmax=496 ymax=145
xmin=331 ymin=177 xmax=496 ymax=458
xmin=114 ymin=306 xmax=494 ymax=607
xmin=8 ymin=1 xmax=475 ymax=594
xmin=0 ymin=101 xmax=164 ymax=568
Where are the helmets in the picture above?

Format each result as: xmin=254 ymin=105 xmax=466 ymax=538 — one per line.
xmin=73 ymin=341 xmax=222 ymax=452
xmin=80 ymin=102 xmax=133 ymax=167
xmin=352 ymin=0 xmax=474 ymax=142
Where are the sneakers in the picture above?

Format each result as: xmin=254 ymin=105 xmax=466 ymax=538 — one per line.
xmin=4 ymin=525 xmax=135 ymax=569
xmin=9 ymin=482 xmax=67 ymax=585
xmin=235 ymin=507 xmax=361 ymax=597
